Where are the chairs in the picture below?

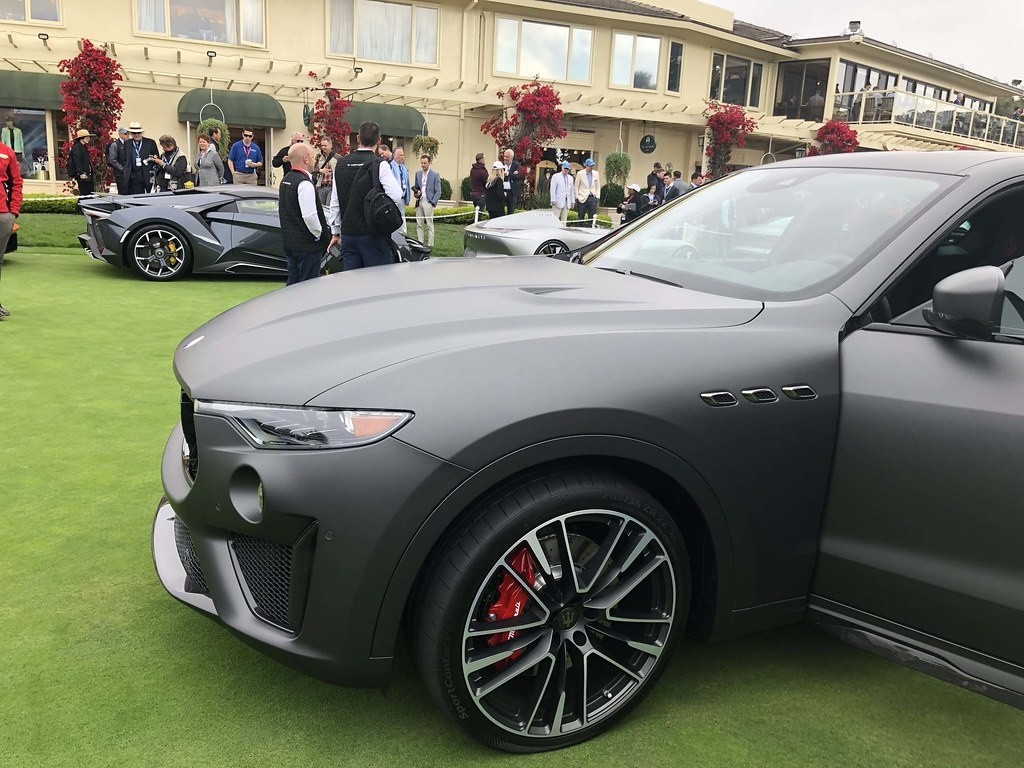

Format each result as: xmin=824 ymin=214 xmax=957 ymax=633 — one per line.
xmin=768 ymin=201 xmax=863 ymax=265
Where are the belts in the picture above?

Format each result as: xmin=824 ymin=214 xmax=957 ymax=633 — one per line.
xmin=400 ymin=197 xmax=405 ymax=199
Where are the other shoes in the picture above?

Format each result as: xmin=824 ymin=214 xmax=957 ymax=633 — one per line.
xmin=0 ymin=306 xmax=10 ymax=317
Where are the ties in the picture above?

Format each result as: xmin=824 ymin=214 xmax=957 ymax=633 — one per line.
xmin=398 ymin=165 xmax=403 ymax=191
xmin=565 ymin=175 xmax=568 ymax=188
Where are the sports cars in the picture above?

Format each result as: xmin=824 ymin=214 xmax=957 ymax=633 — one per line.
xmin=77 ymin=184 xmax=433 ymax=282
xmin=460 ymin=208 xmax=699 ymax=271
xmin=149 ymin=151 xmax=1024 ymax=754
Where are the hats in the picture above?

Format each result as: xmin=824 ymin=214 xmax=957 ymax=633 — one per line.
xmin=585 ymin=159 xmax=595 ymax=166
xmin=128 ymin=122 xmax=144 ymax=132
xmin=654 ymin=163 xmax=662 ymax=168
xmin=563 ymin=163 xmax=570 ymax=169
xmin=73 ymin=129 xmax=96 ymax=139
xmin=626 ymin=184 xmax=641 ymax=193
xmin=5 ymin=116 xmax=15 ymax=122
xmin=118 ymin=128 xmax=128 ymax=134
xmin=492 ymin=161 xmax=506 ymax=170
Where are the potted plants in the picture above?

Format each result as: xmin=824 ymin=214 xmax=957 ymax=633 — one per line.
xmin=412 ymin=135 xmax=443 ymax=165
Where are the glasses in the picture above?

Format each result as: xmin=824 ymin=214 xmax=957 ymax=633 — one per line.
xmin=123 ymin=132 xmax=128 ymax=135
xmin=131 ymin=132 xmax=141 ymax=135
xmin=294 ymin=140 xmax=303 ymax=143
xmin=243 ymin=134 xmax=253 ymax=138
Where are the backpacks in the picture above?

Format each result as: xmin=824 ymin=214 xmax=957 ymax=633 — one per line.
xmin=362 ymin=159 xmax=403 ymax=241
xmin=66 ymin=147 xmax=77 ymax=178
xmin=106 ymin=141 xmax=119 ymax=167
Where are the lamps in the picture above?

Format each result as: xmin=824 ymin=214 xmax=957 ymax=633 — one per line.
xmin=848 ymin=20 xmax=860 ymax=31
xmin=795 ymin=148 xmax=806 ymax=158
xmin=354 ymin=67 xmax=363 ymax=73
xmin=1012 ymin=79 xmax=1022 ymax=85
xmin=698 ymin=134 xmax=705 ymax=147
xmin=207 ymin=51 xmax=216 ymax=57
xmin=38 ymin=33 xmax=49 ymax=39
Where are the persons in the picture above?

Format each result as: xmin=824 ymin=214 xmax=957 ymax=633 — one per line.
xmin=788 ymin=95 xmax=799 ymax=106
xmin=271 ymin=122 xmax=442 ymax=285
xmin=0 ymin=116 xmax=24 ymax=161
xmin=122 ymin=122 xmax=160 ymax=194
xmin=109 ymin=129 xmax=129 ymax=194
xmin=574 ymin=159 xmax=600 ymax=227
xmin=152 ymin=135 xmax=187 ymax=191
xmin=954 ymin=93 xmax=963 ymax=105
xmin=616 ymin=162 xmax=702 ymax=225
xmin=854 ymin=82 xmax=883 ymax=121
xmin=0 ymin=142 xmax=24 ymax=318
xmin=194 ymin=128 xmax=263 ymax=188
xmin=1004 ymin=107 xmax=1024 ymax=146
xmin=550 ymin=163 xmax=575 ymax=226
xmin=888 ymin=86 xmax=897 ymax=96
xmin=809 ymin=89 xmax=824 ymax=105
xmin=71 ymin=129 xmax=96 ymax=214
xmin=470 ymin=148 xmax=524 ymax=219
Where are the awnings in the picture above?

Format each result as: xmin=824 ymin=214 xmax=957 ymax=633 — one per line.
xmin=339 ymin=101 xmax=428 ymax=138
xmin=0 ymin=69 xmax=78 ymax=110
xmin=178 ymin=88 xmax=286 ymax=129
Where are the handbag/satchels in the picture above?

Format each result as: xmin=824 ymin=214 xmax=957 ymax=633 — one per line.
xmin=411 ymin=186 xmax=421 ymax=198
